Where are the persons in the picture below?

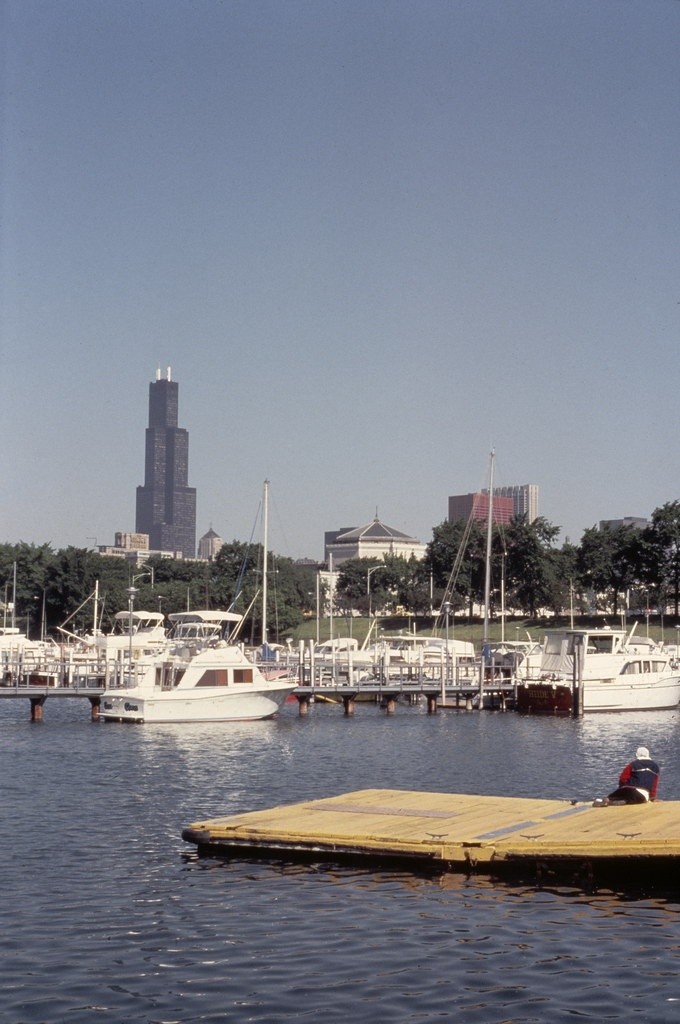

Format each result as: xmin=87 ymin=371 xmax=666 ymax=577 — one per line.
xmin=593 ymin=746 xmax=663 ymax=808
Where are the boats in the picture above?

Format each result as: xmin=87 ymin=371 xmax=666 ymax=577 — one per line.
xmin=0 ymin=561 xmax=680 ymax=702
xmin=96 ymin=646 xmax=298 ymax=723
xmin=522 ymin=655 xmax=680 ymax=711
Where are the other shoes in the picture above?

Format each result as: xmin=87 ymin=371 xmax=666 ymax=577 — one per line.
xmin=594 ymin=799 xmax=627 ymax=808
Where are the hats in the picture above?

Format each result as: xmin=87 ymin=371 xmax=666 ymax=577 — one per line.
xmin=636 ymin=747 xmax=650 ymax=760
